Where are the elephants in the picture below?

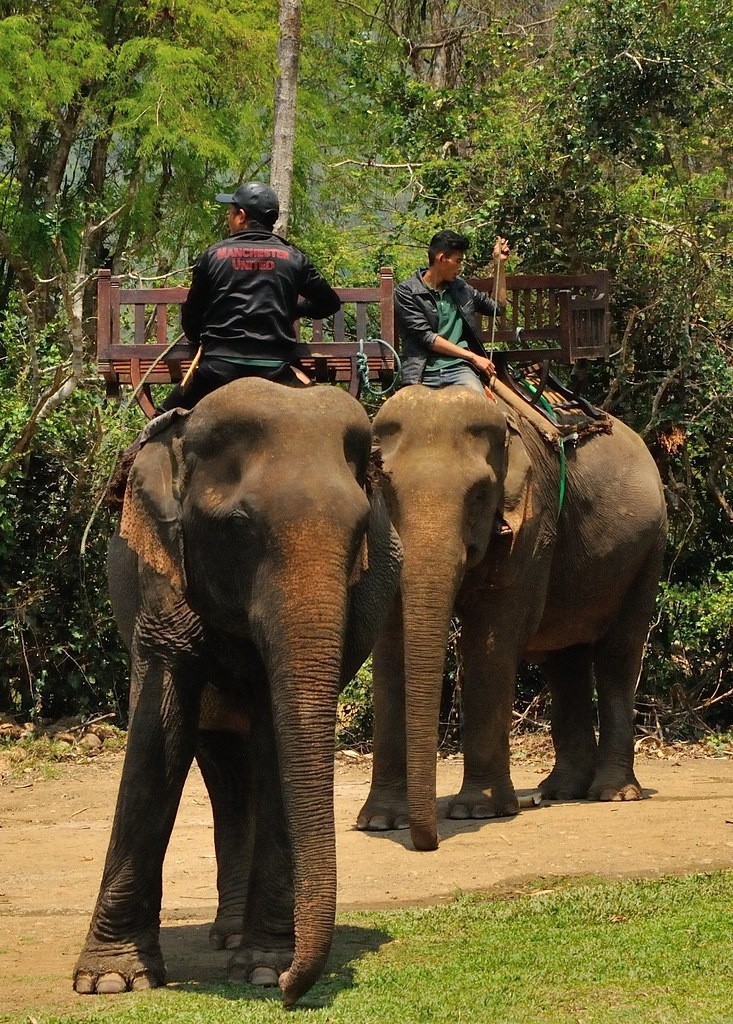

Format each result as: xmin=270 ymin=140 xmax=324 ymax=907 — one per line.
xmin=71 ymin=375 xmax=668 ymax=1014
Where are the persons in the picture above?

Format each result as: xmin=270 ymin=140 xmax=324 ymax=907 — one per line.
xmin=395 ymin=230 xmax=509 ymax=397
xmin=151 ymin=181 xmax=340 ymax=420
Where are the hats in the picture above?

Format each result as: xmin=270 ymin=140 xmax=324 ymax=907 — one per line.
xmin=215 ymin=181 xmax=279 ymax=225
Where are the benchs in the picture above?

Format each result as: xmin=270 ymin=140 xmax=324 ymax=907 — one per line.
xmin=96 ymin=266 xmax=395 ymax=384
xmin=401 ymin=267 xmax=611 ymax=364
